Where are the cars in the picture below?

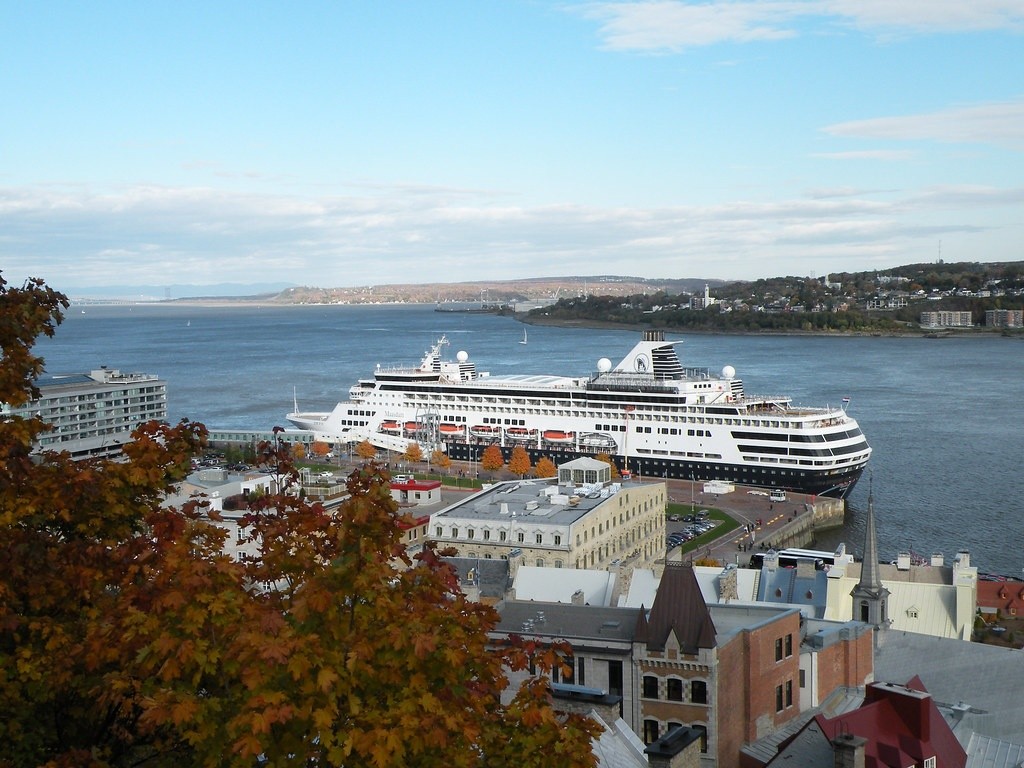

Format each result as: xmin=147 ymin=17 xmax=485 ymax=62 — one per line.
xmin=189 ymin=450 xmax=279 ymax=475
xmin=665 ymin=509 xmax=716 ymax=554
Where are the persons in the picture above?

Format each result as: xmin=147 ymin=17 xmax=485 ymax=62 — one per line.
xmin=459 ymin=469 xmax=479 ymax=478
xmin=747 ymin=523 xmax=755 ymax=533
xmin=738 ymin=540 xmax=772 ymax=552
xmin=759 ymin=518 xmax=763 ymax=526
xmin=794 ymin=509 xmax=797 ymax=516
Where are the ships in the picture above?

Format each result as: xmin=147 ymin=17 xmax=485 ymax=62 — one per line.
xmin=283 ymin=326 xmax=872 ymax=498
